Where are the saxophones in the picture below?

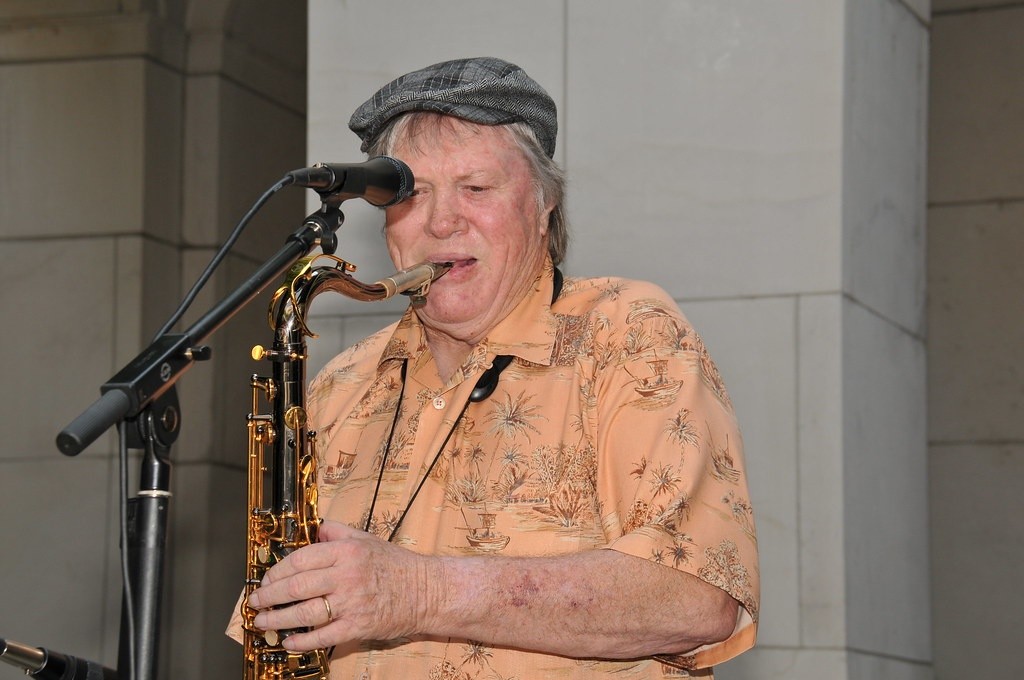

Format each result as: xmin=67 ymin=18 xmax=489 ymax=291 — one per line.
xmin=238 ymin=253 xmax=453 ymax=680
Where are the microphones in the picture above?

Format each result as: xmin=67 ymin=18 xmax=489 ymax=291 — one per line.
xmin=286 ymin=155 xmax=416 ymax=209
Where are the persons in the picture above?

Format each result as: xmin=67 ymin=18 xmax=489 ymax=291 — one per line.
xmin=228 ymin=57 xmax=759 ymax=680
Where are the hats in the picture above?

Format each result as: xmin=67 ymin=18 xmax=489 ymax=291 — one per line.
xmin=349 ymin=57 xmax=558 ymax=160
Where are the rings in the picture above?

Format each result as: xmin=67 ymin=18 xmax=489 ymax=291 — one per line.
xmin=322 ymin=596 xmax=333 ymax=623
